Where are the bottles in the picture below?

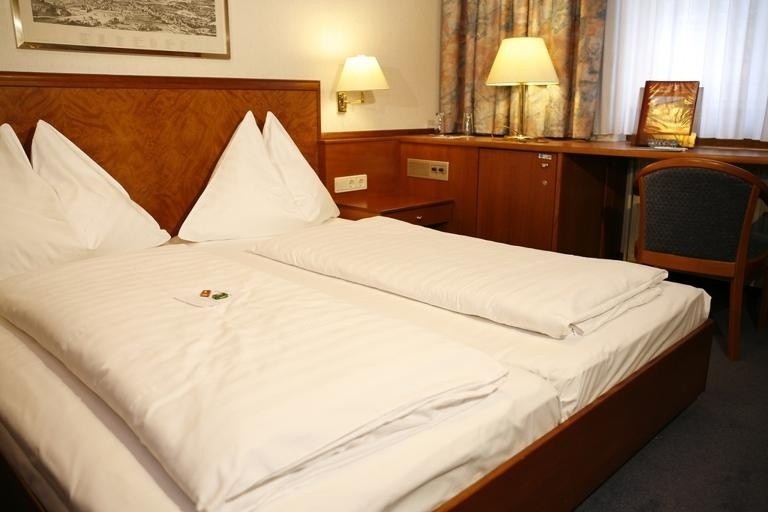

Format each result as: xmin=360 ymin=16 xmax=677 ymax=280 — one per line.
xmin=433 ymin=113 xmax=441 ymax=132
xmin=462 ymin=112 xmax=473 ymax=134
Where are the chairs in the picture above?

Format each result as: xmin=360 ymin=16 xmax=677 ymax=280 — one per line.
xmin=630 ymin=157 xmax=767 ymax=362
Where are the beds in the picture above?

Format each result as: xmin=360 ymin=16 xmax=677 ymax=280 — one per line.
xmin=0 ymin=69 xmax=712 ymax=510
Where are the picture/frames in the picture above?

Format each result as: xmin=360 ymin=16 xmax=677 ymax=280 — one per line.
xmin=8 ymin=0 xmax=233 ymax=62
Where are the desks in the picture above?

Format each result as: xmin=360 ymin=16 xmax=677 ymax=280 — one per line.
xmin=400 ymin=135 xmax=768 ymax=337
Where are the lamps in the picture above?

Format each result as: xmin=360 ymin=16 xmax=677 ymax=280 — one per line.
xmin=336 ymin=52 xmax=391 ymax=114
xmin=485 ymin=36 xmax=561 ymax=141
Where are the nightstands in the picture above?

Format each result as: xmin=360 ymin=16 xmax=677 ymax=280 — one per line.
xmin=331 ymin=192 xmax=455 ymax=235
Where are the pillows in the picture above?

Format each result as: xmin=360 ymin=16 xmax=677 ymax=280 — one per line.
xmin=178 ymin=108 xmax=340 ymax=241
xmin=1 ymin=118 xmax=170 ymax=281
